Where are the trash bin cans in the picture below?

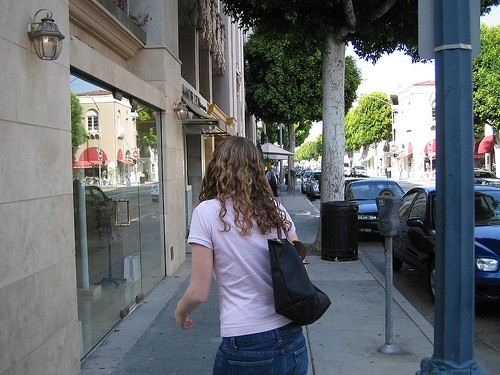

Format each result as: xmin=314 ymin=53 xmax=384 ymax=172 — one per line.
xmin=320 ymin=201 xmax=359 ymax=262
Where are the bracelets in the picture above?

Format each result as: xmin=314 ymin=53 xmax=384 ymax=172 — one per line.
xmin=177 ymin=301 xmax=188 ymax=315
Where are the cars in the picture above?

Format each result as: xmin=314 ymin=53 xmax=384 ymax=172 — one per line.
xmin=343 ymin=175 xmax=367 ymax=198
xmin=344 ymin=163 xmax=350 ymax=177
xmin=306 ymin=171 xmax=321 ymax=198
xmin=383 ymin=185 xmax=500 ymax=305
xmin=345 ymin=178 xmax=406 ymax=234
xmin=351 ymin=166 xmax=366 ymax=177
xmin=300 ymin=171 xmax=312 ymax=192
xmin=474 ymin=171 xmax=500 ymax=188
xmin=74 ymin=185 xmax=119 ymax=258
xmin=151 ymin=182 xmax=160 ymax=201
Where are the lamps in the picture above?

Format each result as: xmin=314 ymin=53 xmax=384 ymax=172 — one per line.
xmin=27 ymin=8 xmax=65 ymax=60
xmin=173 ymin=98 xmax=188 ymax=120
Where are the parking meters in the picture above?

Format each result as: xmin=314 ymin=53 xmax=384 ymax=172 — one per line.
xmin=291 ymin=169 xmax=297 ymax=197
xmin=374 ymin=188 xmax=402 ymax=353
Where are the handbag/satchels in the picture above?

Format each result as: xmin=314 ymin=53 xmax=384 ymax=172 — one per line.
xmin=267 ymin=226 xmax=331 ymax=326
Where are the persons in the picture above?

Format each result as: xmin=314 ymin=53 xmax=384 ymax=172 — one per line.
xmin=144 ymin=168 xmax=148 ymax=178
xmin=126 ymin=170 xmax=131 ymax=185
xmin=93 ymin=177 xmax=97 ymax=185
xmin=282 ymin=166 xmax=286 ymax=180
xmin=273 ymin=166 xmax=279 ymax=180
xmin=73 ymin=175 xmax=79 ymax=190
xmin=174 ymin=136 xmax=308 ymax=375
xmin=266 ymin=167 xmax=280 ymax=197
xmin=351 ymin=168 xmax=355 ymax=177
xmin=295 ymin=164 xmax=311 ymax=177
xmin=375 ymin=166 xmax=381 ymax=177
xmin=284 ymin=167 xmax=289 ymax=186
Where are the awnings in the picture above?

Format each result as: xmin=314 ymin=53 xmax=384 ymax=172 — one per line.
xmin=428 ymin=152 xmax=436 ymax=160
xmin=396 ymin=153 xmax=412 ymax=161
xmin=72 ymin=160 xmax=110 ymax=168
xmin=117 ymin=159 xmax=134 ymax=167
